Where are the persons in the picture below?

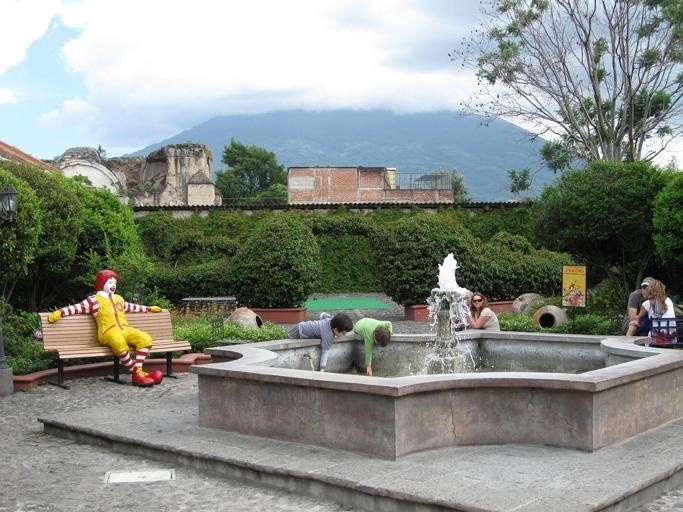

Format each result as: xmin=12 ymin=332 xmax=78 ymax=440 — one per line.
xmin=637 ymin=280 xmax=677 ymax=348
xmin=621 ymin=277 xmax=656 ymax=337
xmin=464 ymin=292 xmax=500 ymax=331
xmin=350 ymin=316 xmax=394 ymax=376
xmin=46 ymin=268 xmax=165 ymax=388
xmin=286 ymin=312 xmax=353 ymax=372
xmin=450 ymin=291 xmax=473 ymax=330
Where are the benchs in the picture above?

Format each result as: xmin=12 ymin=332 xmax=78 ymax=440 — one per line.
xmin=181 ymin=297 xmax=237 ymax=314
xmin=38 ymin=308 xmax=192 ymax=389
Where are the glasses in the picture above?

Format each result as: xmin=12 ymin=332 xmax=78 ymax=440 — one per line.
xmin=472 ymin=297 xmax=483 ymax=303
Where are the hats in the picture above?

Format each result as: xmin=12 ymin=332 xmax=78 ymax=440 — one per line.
xmin=640 ymin=276 xmax=656 ymax=287
